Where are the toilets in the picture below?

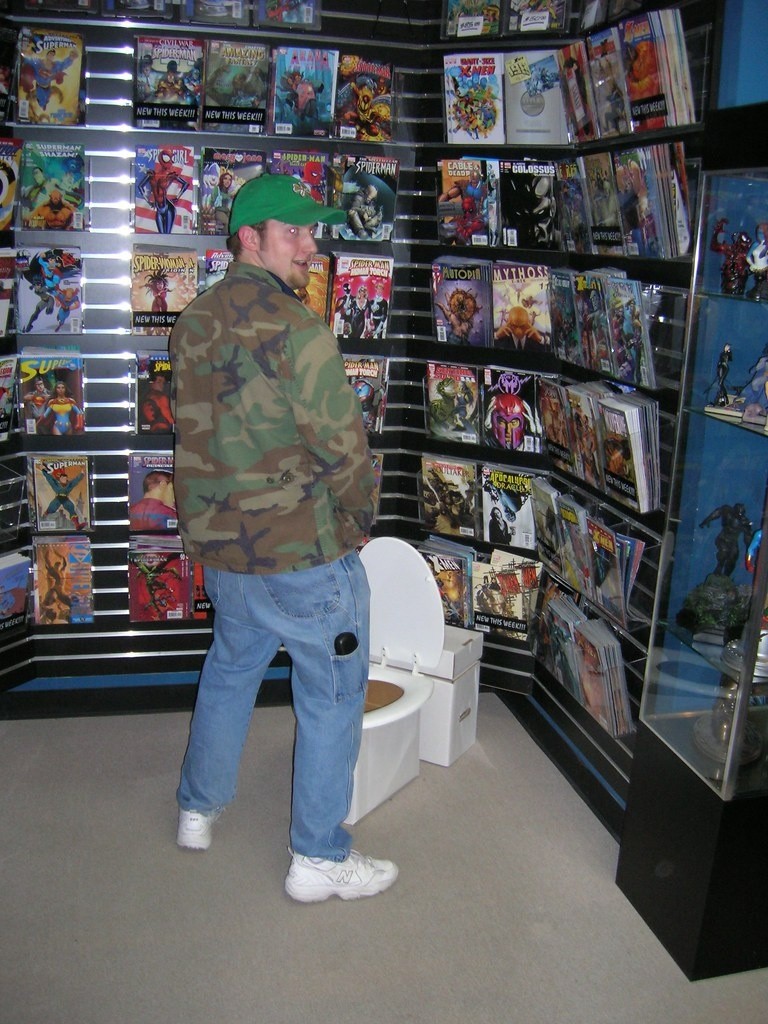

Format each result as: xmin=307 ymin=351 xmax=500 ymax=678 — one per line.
xmin=340 ymin=536 xmax=444 ymax=827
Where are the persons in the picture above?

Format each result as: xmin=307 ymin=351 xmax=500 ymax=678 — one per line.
xmin=337 ymin=283 xmax=483 ymax=346
xmin=699 ymin=503 xmax=753 ymax=577
xmin=169 ymin=174 xmax=401 ymax=902
xmin=144 ymin=370 xmax=174 ymax=434
xmin=348 ymin=186 xmax=383 ymax=240
xmin=746 ymin=530 xmax=762 ymax=573
xmin=707 ymin=344 xmax=732 ymax=407
xmin=489 ymin=507 xmax=516 ymax=546
xmin=747 ymin=224 xmax=768 ymax=287
xmin=137 ymin=54 xmax=202 ymax=106
xmin=712 ymin=675 xmax=738 ymax=741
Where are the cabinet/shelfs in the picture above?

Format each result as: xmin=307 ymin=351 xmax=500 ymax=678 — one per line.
xmin=615 ymin=167 xmax=768 ymax=980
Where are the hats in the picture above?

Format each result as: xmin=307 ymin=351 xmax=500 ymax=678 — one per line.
xmin=230 ymin=173 xmax=347 ymax=226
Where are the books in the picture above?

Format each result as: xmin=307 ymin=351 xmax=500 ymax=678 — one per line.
xmin=129 ymin=37 xmax=398 ymax=624
xmin=432 ymin=0 xmax=696 ymax=393
xmin=5 ymin=0 xmax=323 ymax=31
xmin=416 ymin=360 xmax=662 ymax=738
xmin=0 ymin=29 xmax=94 ymax=631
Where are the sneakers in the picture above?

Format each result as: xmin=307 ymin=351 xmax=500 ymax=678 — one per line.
xmin=176 ymin=805 xmax=225 ymax=849
xmin=285 ymin=845 xmax=399 ymax=902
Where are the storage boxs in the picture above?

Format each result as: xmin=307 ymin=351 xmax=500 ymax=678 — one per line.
xmin=365 ymin=621 xmax=484 ymax=766
